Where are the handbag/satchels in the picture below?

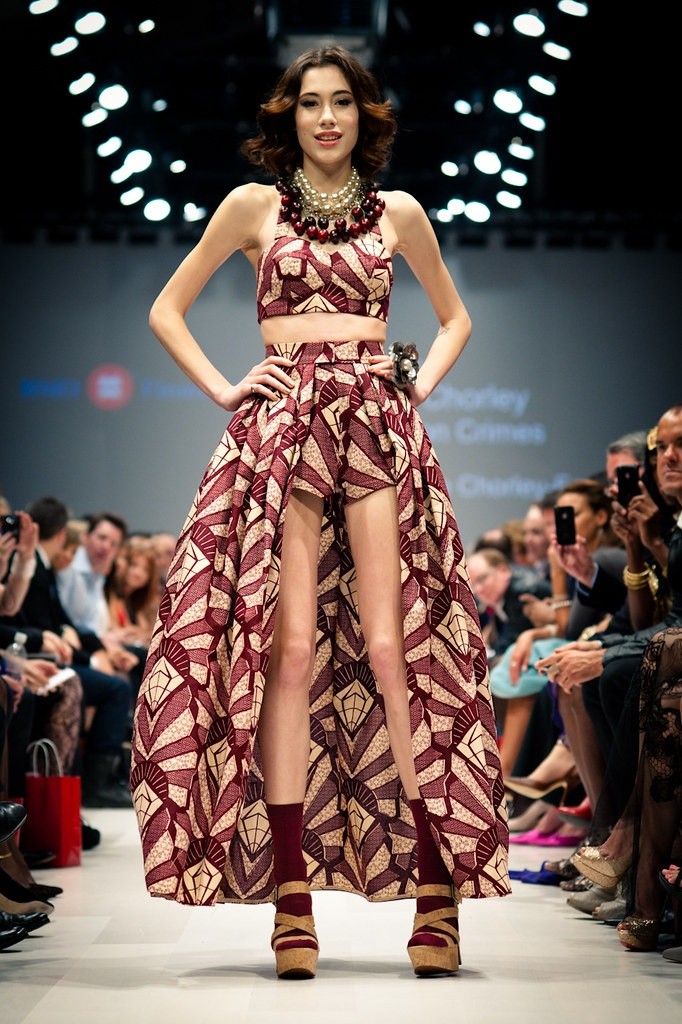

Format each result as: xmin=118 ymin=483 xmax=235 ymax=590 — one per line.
xmin=21 ymin=739 xmax=82 ymax=869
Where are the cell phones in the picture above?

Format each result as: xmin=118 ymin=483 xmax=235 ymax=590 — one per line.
xmin=0 ymin=515 xmax=22 ymax=536
xmin=554 ymin=506 xmax=576 ymax=546
xmin=616 ymin=465 xmax=642 ymax=518
xmin=37 ymin=668 xmax=76 ymax=695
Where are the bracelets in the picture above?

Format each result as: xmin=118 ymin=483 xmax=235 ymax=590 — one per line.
xmin=552 ymin=600 xmax=572 ymax=610
xmin=624 ymin=564 xmax=650 ymax=589
xmin=11 ymin=554 xmax=36 ymax=576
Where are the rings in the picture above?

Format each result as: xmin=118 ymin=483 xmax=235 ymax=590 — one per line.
xmin=551 ymin=665 xmax=559 ymax=673
xmin=511 ymin=662 xmax=516 ymax=666
xmin=251 ymin=384 xmax=256 ymax=393
xmin=388 ymin=341 xmax=420 ymax=390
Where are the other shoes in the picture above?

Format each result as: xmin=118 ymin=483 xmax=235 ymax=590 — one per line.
xmin=0 ymin=834 xmax=63 ymax=913
xmin=79 ymin=822 xmax=99 ymax=850
xmin=498 ymin=769 xmax=682 ymax=962
xmin=80 ymin=776 xmax=132 ymax=806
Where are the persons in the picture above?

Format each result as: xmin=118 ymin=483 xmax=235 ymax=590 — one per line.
xmin=466 ymin=406 xmax=682 ymax=962
xmin=0 ymin=493 xmax=178 ymax=949
xmin=131 ymin=44 xmax=513 ymax=981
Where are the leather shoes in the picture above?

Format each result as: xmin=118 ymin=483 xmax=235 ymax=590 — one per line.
xmin=0 ymin=923 xmax=27 ymax=952
xmin=0 ymin=799 xmax=28 ymax=845
xmin=0 ymin=909 xmax=50 ymax=932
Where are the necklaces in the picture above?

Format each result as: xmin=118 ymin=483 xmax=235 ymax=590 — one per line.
xmin=276 ymin=165 xmax=386 ymax=244
xmin=547 ymin=624 xmax=556 ymax=637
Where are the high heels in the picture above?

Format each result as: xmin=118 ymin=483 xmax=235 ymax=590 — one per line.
xmin=270 ymin=881 xmax=320 ymax=979
xmin=406 ymin=883 xmax=462 ymax=975
